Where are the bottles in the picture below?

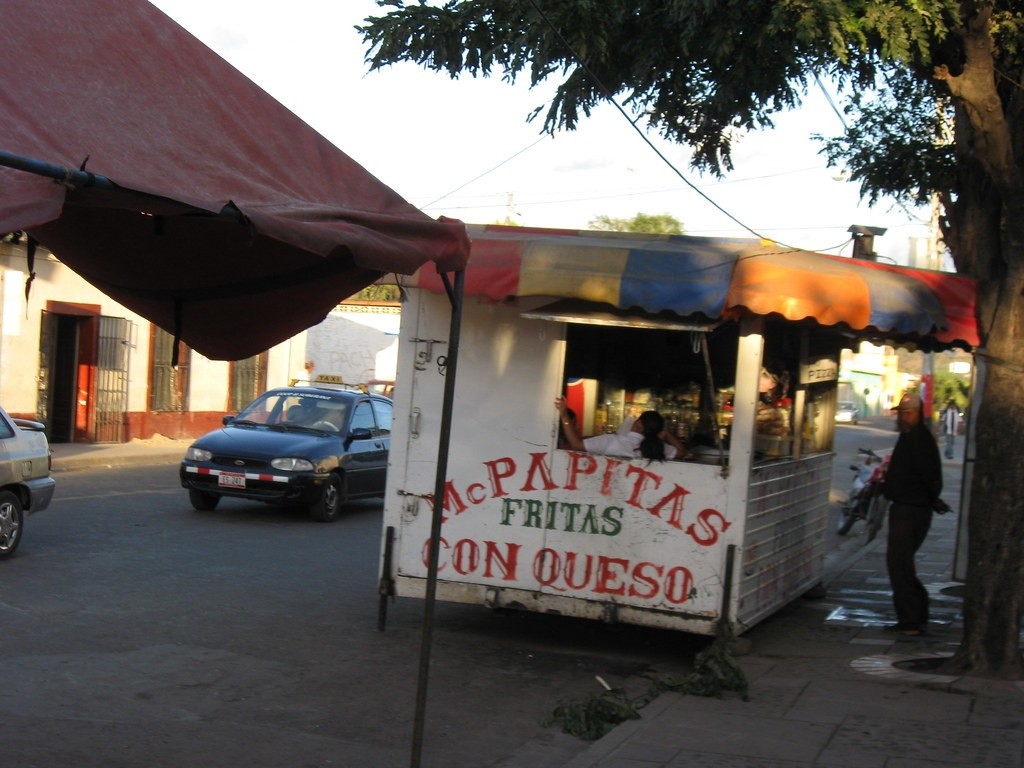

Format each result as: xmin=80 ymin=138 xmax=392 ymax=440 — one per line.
xmin=677 ymin=411 xmax=689 ymax=442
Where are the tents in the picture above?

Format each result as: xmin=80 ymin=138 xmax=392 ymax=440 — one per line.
xmin=0 ymin=0 xmax=473 ymax=768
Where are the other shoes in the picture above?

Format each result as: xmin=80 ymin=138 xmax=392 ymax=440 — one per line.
xmin=885 ymin=622 xmax=923 ymax=636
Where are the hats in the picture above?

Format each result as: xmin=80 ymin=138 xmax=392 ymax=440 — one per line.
xmin=890 ymin=393 xmax=923 ymax=412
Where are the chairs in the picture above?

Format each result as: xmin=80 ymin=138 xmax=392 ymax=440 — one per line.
xmin=286 ymin=405 xmax=306 ymax=426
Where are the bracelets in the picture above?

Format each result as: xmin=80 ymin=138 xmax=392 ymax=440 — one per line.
xmin=563 ymin=421 xmax=570 ymax=425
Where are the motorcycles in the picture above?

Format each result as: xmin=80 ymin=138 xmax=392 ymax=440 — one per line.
xmin=836 ymin=447 xmax=891 ymax=546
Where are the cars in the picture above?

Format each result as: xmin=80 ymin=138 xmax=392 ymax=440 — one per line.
xmin=178 ymin=373 xmax=395 ymax=523
xmin=834 ymin=401 xmax=860 ymax=426
xmin=0 ymin=403 xmax=55 ymax=560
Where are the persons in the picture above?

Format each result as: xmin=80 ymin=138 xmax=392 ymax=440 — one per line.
xmin=882 ymin=394 xmax=951 ymax=634
xmin=943 ymin=400 xmax=958 ymax=459
xmin=555 ymin=395 xmax=687 ymax=461
